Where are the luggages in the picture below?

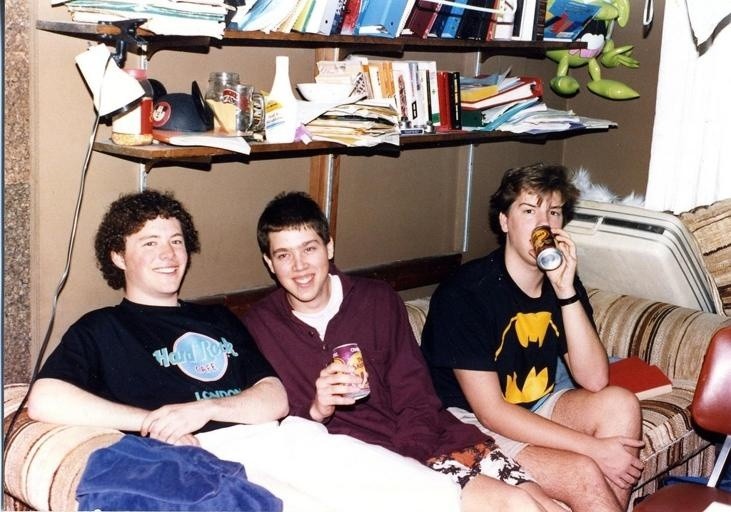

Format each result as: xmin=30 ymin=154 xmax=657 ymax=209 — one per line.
xmin=561 ymin=198 xmax=725 ymax=315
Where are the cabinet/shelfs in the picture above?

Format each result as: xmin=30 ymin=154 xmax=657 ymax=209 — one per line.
xmin=35 ymin=20 xmax=607 ymax=170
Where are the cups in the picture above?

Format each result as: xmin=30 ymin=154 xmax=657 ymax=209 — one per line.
xmin=205 ymin=71 xmax=268 ymax=138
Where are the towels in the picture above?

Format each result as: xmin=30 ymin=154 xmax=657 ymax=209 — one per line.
xmin=76 ymin=434 xmax=283 ymax=511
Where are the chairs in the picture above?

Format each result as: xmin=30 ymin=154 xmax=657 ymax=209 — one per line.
xmin=633 ymin=327 xmax=731 ymax=511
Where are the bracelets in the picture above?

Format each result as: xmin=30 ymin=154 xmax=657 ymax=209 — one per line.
xmin=555 ymin=293 xmax=580 ymax=306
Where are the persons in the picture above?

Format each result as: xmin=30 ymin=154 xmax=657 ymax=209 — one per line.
xmin=236 ymin=192 xmax=566 ymax=512
xmin=419 ymin=160 xmax=646 ymax=512
xmin=26 ymin=190 xmax=463 ymax=512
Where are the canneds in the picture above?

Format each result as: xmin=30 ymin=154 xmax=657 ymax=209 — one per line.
xmin=331 ymin=342 xmax=372 ymax=402
xmin=532 ymin=225 xmax=562 ymax=272
xmin=203 ymin=71 xmax=266 ymax=134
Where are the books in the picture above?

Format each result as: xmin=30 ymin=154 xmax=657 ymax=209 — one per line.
xmin=153 ymin=128 xmax=250 ymax=155
xmin=51 ymin=0 xmax=537 ymax=42
xmin=317 ymin=56 xmax=548 ymax=131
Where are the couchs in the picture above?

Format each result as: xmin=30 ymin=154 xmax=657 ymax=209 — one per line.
xmin=2 ymin=283 xmax=731 ymax=511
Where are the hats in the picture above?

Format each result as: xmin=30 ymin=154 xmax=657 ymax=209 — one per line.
xmin=147 ymin=79 xmax=213 ymax=132
